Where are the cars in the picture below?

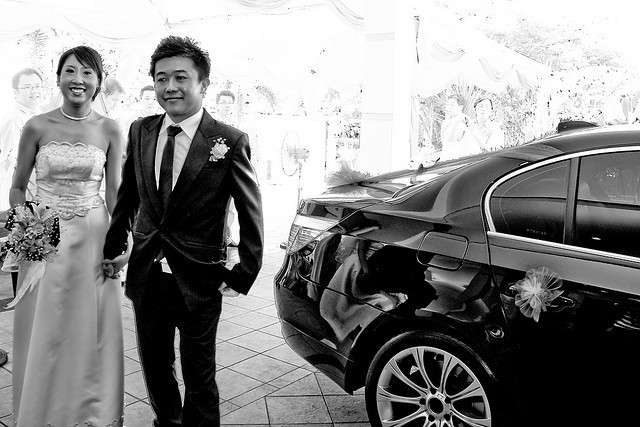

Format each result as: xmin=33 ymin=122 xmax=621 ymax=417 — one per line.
xmin=273 ymin=118 xmax=640 ymax=427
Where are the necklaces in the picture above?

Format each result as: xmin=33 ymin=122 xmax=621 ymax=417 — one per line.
xmin=59 ymin=106 xmax=92 ymax=120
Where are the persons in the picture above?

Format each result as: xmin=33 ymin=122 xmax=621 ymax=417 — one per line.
xmin=215 ymin=89 xmax=239 ymax=246
xmin=102 ymin=35 xmax=264 ymax=425
xmin=472 ymin=98 xmax=502 ymax=148
xmin=441 ymin=94 xmax=477 ymax=160
xmin=136 ymin=86 xmax=161 ymax=114
xmin=9 ymin=44 xmax=133 ymax=427
xmin=95 ymin=78 xmax=127 ymax=118
xmin=0 ymin=67 xmax=43 ymax=299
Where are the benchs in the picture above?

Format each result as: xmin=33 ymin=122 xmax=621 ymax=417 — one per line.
xmin=522 ymin=177 xmax=617 ymax=240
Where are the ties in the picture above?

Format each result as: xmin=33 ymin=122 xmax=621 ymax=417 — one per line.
xmin=158 ymin=125 xmax=181 ymax=212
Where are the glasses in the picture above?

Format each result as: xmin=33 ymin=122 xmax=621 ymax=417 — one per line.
xmin=13 ymin=84 xmax=45 ymax=91
xmin=218 ymin=100 xmax=235 ymax=106
